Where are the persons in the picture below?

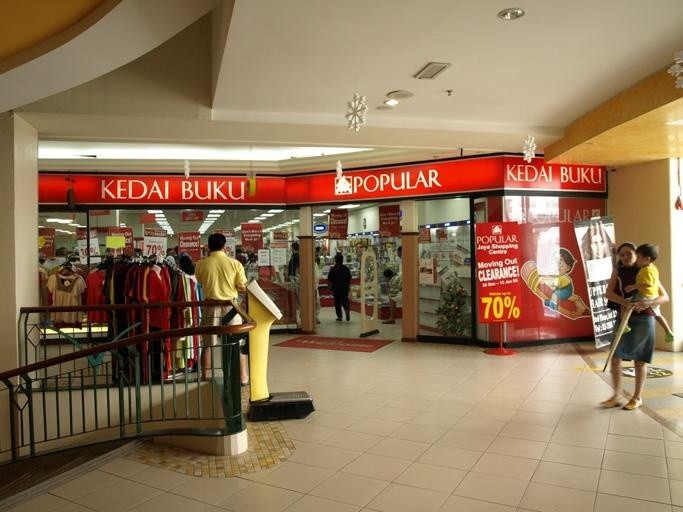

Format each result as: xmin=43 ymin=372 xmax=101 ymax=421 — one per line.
xmin=145 ymin=264 xmax=170 ymax=379
xmin=285 ymin=243 xmax=299 ymax=275
xmin=394 ymin=246 xmax=402 ymax=273
xmin=326 ymin=253 xmax=351 ymax=321
xmin=580 ymin=225 xmax=614 ymax=260
xmin=611 ymin=243 xmax=676 ymax=344
xmin=193 ymin=233 xmax=249 ymax=386
xmin=596 ymin=241 xmax=670 ymax=410
xmin=379 ymin=268 xmax=400 ymax=325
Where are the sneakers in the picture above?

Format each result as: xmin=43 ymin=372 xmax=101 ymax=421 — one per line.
xmin=614 ymin=326 xmax=631 ymax=336
xmin=664 ymin=332 xmax=674 ymax=342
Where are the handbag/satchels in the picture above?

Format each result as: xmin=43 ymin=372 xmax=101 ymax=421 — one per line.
xmin=607 ymin=277 xmax=624 ymax=310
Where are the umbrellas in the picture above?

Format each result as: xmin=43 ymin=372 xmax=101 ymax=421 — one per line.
xmin=600 ymin=288 xmax=638 ymax=372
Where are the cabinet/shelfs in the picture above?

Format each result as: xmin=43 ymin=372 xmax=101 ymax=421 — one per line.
xmin=337 ymin=230 xmax=402 ymax=277
xmin=318 ymin=275 xmax=360 ymax=307
xmin=349 ymin=297 xmax=402 ymax=320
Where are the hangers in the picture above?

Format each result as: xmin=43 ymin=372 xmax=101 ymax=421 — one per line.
xmin=97 ymin=254 xmax=162 ymax=274
xmin=162 ymin=256 xmax=186 ymax=277
xmin=58 ymin=254 xmax=78 ymax=281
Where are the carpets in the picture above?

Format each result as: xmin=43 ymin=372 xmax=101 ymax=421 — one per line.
xmin=272 ymin=334 xmax=395 ymax=352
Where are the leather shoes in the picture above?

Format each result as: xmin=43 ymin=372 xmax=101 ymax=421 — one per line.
xmin=600 ymin=397 xmax=623 ymax=407
xmin=623 ymin=398 xmax=642 ymax=410
